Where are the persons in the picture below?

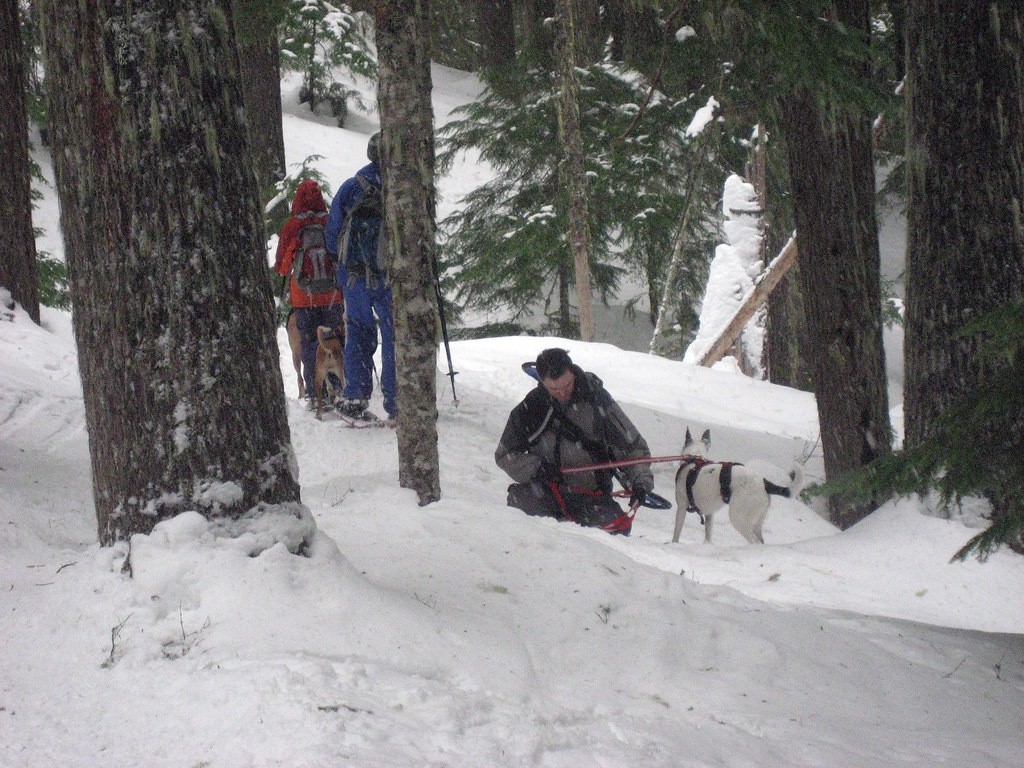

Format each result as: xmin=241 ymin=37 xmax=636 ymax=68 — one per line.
xmin=323 ymin=132 xmax=398 ymax=421
xmin=276 ymin=180 xmax=345 ymax=411
xmin=494 ymin=348 xmax=655 ymax=537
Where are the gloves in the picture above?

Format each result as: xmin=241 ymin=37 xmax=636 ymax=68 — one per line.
xmin=536 ymin=461 xmax=564 ymax=485
xmin=628 ymin=488 xmax=646 ymax=506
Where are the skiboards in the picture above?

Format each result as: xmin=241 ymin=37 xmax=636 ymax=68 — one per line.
xmin=329 ymin=401 xmax=407 ymax=430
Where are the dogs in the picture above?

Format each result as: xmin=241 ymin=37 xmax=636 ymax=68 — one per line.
xmin=671 ymin=426 xmax=806 ymax=546
xmin=286 ymin=307 xmax=352 ymax=420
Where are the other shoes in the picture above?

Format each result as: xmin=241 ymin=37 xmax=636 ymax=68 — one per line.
xmin=334 ymin=398 xmax=384 ymax=429
xmin=388 ymin=410 xmax=398 ymax=430
xmin=311 ymin=397 xmax=334 ymax=412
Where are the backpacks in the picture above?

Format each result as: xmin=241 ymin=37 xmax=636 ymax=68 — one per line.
xmin=287 ymin=212 xmax=339 ymax=311
xmin=335 ymin=173 xmax=387 ymax=290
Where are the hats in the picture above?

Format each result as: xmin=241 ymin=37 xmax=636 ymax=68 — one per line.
xmin=367 ymin=132 xmax=379 ymax=162
